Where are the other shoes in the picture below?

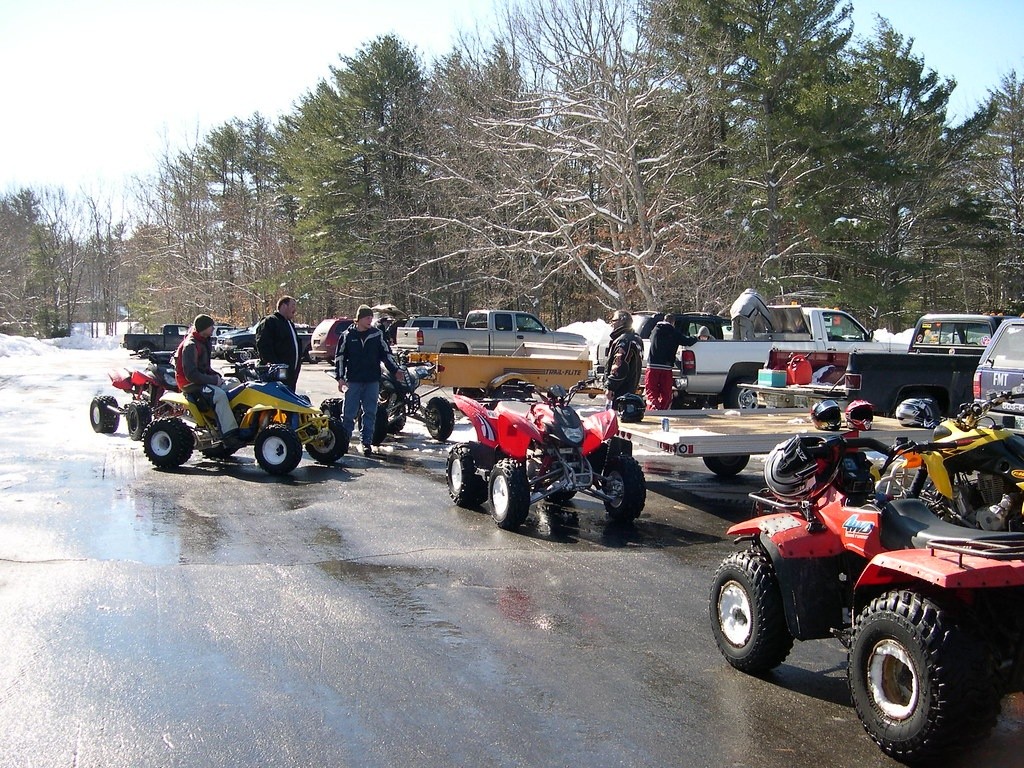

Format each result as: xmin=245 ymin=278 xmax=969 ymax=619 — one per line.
xmin=222 ymin=433 xmax=247 ymax=446
xmin=361 ymin=442 xmax=373 ymax=453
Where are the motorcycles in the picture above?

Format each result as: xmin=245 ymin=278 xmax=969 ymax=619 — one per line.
xmin=707 ymin=390 xmax=1024 ymax=760
xmin=320 ymin=350 xmax=455 ymax=442
xmin=90 ymin=348 xmax=181 ymax=442
xmin=445 ymin=376 xmax=647 ymax=531
xmin=141 ymin=364 xmax=350 ymax=476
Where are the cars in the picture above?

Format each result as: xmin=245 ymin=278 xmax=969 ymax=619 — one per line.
xmin=308 ymin=316 xmax=357 ymax=364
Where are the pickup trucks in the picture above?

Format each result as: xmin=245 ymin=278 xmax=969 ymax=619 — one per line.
xmin=374 ymin=310 xmax=590 ymax=360
xmin=680 ymin=307 xmax=879 ymax=409
xmin=123 ymin=324 xmax=189 ymax=352
xmin=210 ymin=321 xmax=319 ymax=363
xmin=845 ymin=314 xmax=1021 ymax=418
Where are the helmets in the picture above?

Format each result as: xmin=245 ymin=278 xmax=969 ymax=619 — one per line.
xmin=810 ymin=399 xmax=841 ymax=431
xmin=616 ymin=393 xmax=645 ymax=423
xmin=764 ymin=434 xmax=843 ymax=502
xmin=846 ymin=400 xmax=875 ymax=430
xmin=895 ymin=398 xmax=941 ymax=428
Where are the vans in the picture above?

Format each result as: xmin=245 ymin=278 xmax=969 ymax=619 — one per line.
xmin=973 ymin=319 xmax=1024 ymax=435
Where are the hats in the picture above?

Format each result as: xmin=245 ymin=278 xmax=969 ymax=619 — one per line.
xmin=194 ymin=314 xmax=214 ymax=332
xmin=356 ymin=304 xmax=374 ymax=322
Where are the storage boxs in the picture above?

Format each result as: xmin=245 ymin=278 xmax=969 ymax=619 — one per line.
xmin=758 ymin=369 xmax=787 ymax=387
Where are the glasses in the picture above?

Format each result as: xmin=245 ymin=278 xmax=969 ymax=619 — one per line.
xmin=609 ymin=318 xmax=623 ymax=325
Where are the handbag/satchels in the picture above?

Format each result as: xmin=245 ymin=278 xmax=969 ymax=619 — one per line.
xmin=787 ymin=354 xmax=813 ymax=384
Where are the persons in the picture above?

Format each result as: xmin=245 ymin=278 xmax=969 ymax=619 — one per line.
xmin=175 ymin=315 xmax=247 ymax=446
xmin=645 ymin=314 xmax=698 ymax=411
xmin=604 ymin=310 xmax=644 ymax=459
xmin=336 ymin=304 xmax=404 ymax=453
xmin=698 ymin=326 xmax=716 ymax=340
xmin=256 ymin=295 xmax=302 ymax=392
xmin=730 ymin=288 xmax=776 ymax=339
xmin=377 ymin=317 xmax=388 ymax=332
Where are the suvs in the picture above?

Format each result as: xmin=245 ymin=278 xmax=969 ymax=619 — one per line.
xmin=595 ymin=311 xmax=733 ymax=388
